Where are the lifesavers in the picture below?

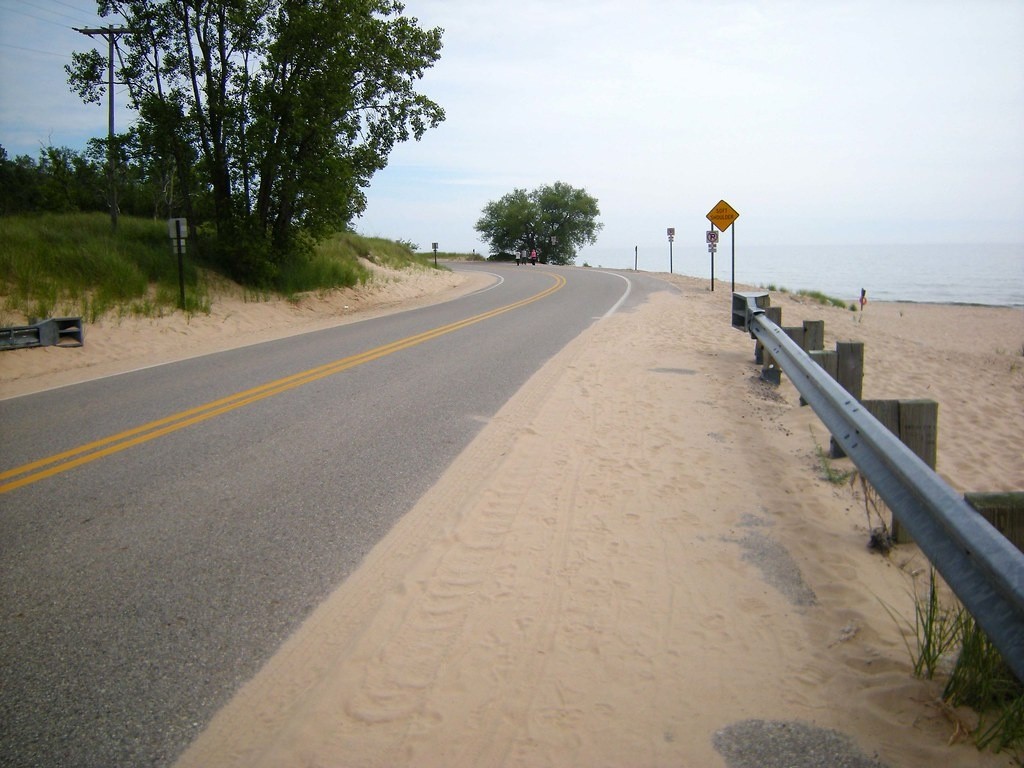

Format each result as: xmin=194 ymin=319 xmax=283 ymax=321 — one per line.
xmin=860 ymin=297 xmax=867 ymax=305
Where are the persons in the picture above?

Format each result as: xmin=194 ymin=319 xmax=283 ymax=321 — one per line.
xmin=530 ymin=249 xmax=537 ymax=265
xmin=521 ymin=248 xmax=527 ymax=265
xmin=514 ymin=250 xmax=521 ymax=266
